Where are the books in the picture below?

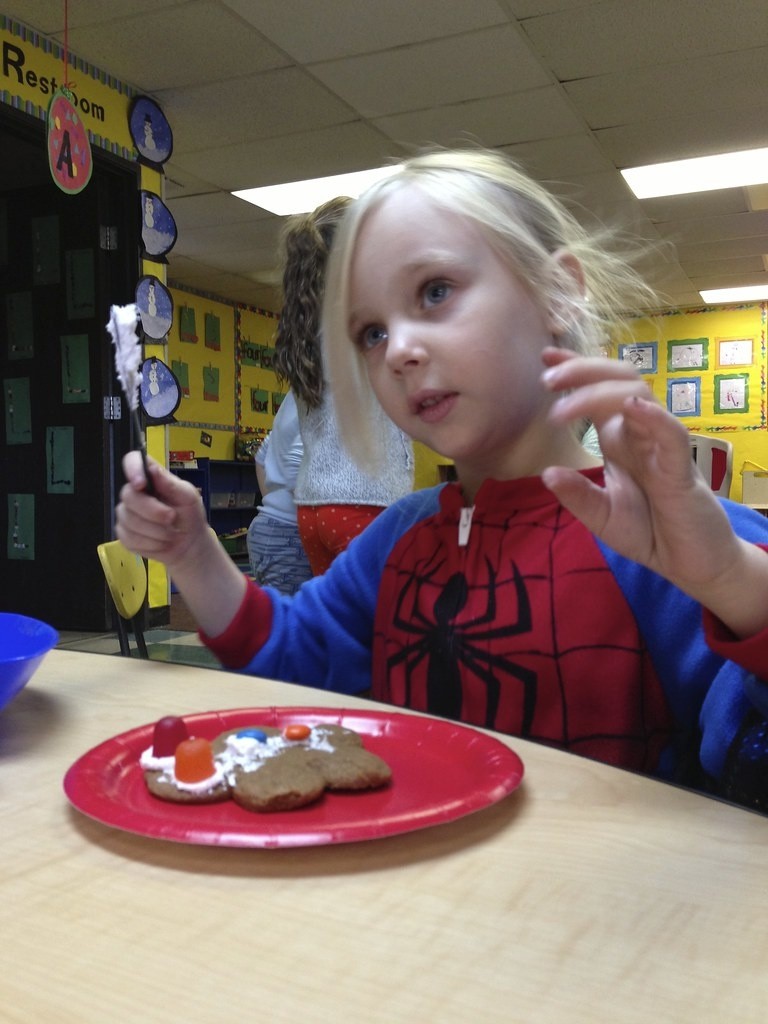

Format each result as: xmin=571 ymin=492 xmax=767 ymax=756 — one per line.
xmin=169 ymin=451 xmax=199 ymax=469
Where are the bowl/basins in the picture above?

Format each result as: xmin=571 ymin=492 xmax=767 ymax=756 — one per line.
xmin=0 ymin=613 xmax=58 ymax=712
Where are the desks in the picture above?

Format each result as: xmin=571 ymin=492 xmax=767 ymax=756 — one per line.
xmin=0 ymin=649 xmax=768 ymax=1024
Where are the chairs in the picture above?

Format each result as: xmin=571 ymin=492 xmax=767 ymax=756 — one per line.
xmin=97 ymin=539 xmax=150 ymax=660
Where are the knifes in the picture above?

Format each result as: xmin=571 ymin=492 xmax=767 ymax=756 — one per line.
xmin=111 ymin=306 xmax=160 ymax=497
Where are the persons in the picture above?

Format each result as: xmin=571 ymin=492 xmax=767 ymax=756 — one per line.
xmin=244 ymin=187 xmax=419 ymax=609
xmin=113 ymin=146 xmax=765 ymax=815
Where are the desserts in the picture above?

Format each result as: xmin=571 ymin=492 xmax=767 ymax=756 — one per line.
xmin=144 ymin=723 xmax=393 ymax=812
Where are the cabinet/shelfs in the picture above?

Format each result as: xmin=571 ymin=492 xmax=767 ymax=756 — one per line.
xmin=170 ymin=457 xmax=263 ymax=558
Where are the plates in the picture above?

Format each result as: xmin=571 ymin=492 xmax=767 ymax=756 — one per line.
xmin=64 ymin=708 xmax=525 ymax=847
